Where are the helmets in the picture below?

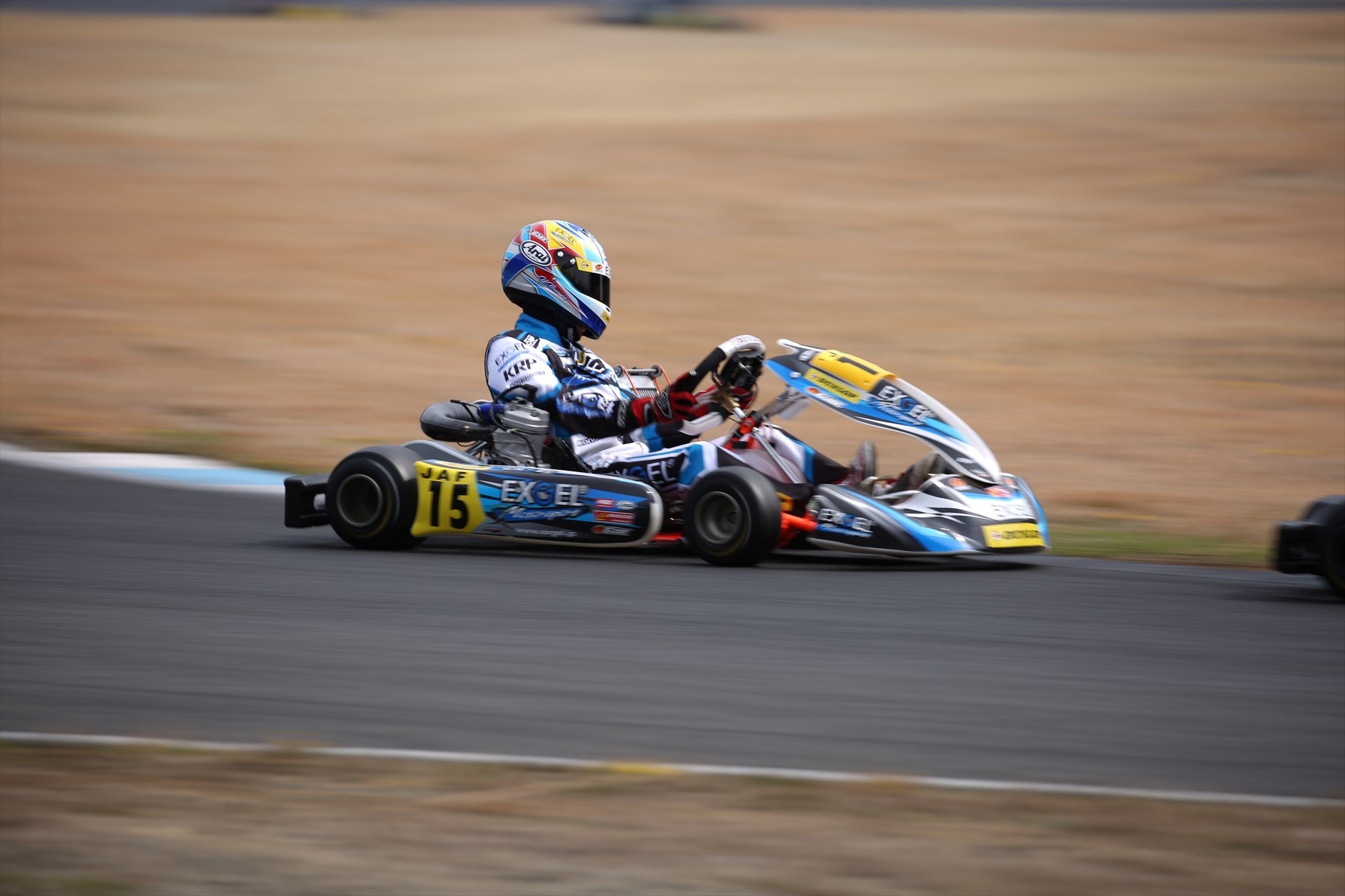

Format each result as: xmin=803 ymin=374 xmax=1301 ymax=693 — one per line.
xmin=500 ymin=219 xmax=611 ymax=341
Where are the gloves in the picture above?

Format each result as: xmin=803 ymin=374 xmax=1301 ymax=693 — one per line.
xmin=695 ymin=381 xmax=756 ymax=417
xmin=630 ymin=369 xmax=700 ymax=428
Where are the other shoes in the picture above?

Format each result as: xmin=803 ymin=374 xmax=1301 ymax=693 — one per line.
xmin=839 ymin=439 xmax=877 ymax=495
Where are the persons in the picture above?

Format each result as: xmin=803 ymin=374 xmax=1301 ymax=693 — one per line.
xmin=484 ymin=219 xmax=941 ymax=503
